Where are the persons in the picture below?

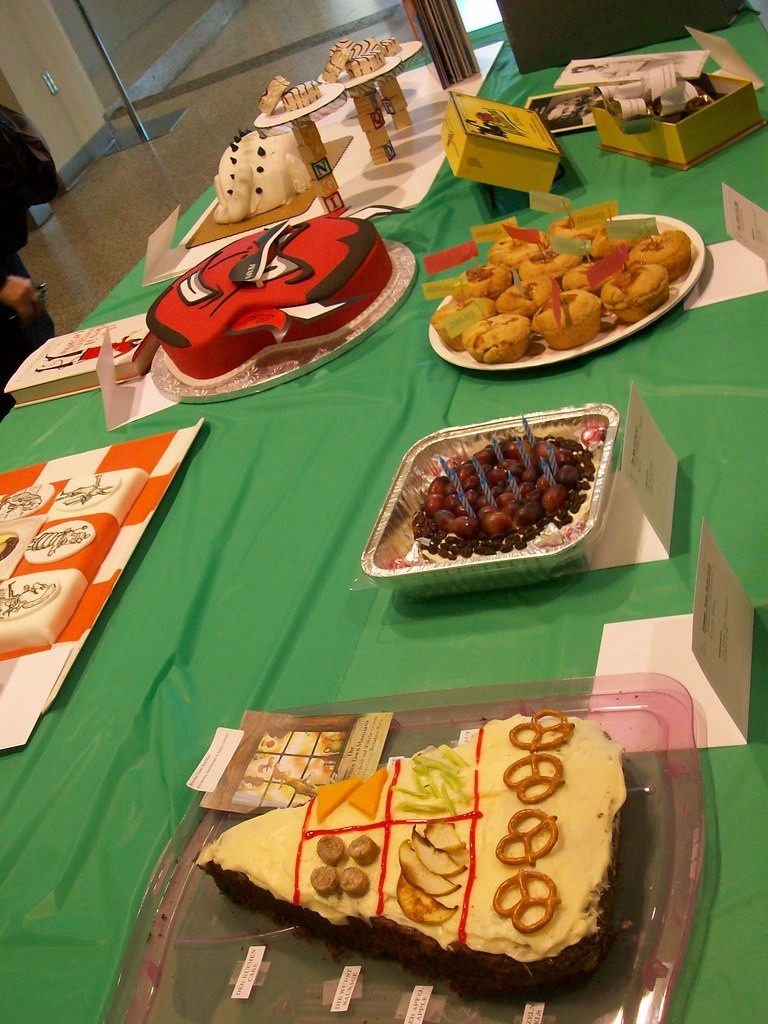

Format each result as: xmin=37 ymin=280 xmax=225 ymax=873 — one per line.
xmin=546 ymin=102 xmax=591 ymax=125
xmin=0 ymin=105 xmax=58 ymax=420
xmin=571 ymin=61 xmax=630 ymax=76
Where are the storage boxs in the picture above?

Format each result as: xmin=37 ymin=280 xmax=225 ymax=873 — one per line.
xmin=590 ymin=73 xmax=763 ymax=165
xmin=441 ymin=92 xmax=564 ymax=194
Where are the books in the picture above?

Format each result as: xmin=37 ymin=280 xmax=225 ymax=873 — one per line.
xmin=4 ymin=313 xmax=159 ymax=407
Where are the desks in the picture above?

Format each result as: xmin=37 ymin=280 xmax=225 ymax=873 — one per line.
xmin=0 ymin=16 xmax=768 ymax=1024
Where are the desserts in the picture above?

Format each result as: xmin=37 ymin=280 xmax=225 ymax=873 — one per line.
xmin=322 ymin=37 xmax=401 ymax=83
xmin=257 ymin=75 xmax=322 ymax=116
xmin=429 ymin=214 xmax=693 ymax=365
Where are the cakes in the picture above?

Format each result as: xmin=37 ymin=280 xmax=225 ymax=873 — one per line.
xmin=144 ymin=204 xmax=412 ymax=387
xmin=412 ymin=434 xmax=596 ymax=564
xmin=0 ymin=467 xmax=150 ymax=660
xmin=194 ymin=708 xmax=626 ymax=999
xmin=212 ymin=125 xmax=311 ymax=224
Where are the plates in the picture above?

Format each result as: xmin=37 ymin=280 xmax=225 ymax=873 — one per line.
xmin=429 ymin=214 xmax=706 ymax=371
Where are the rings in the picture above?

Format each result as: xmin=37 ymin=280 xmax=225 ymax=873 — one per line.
xmin=31 ymin=293 xmax=47 ymax=303
